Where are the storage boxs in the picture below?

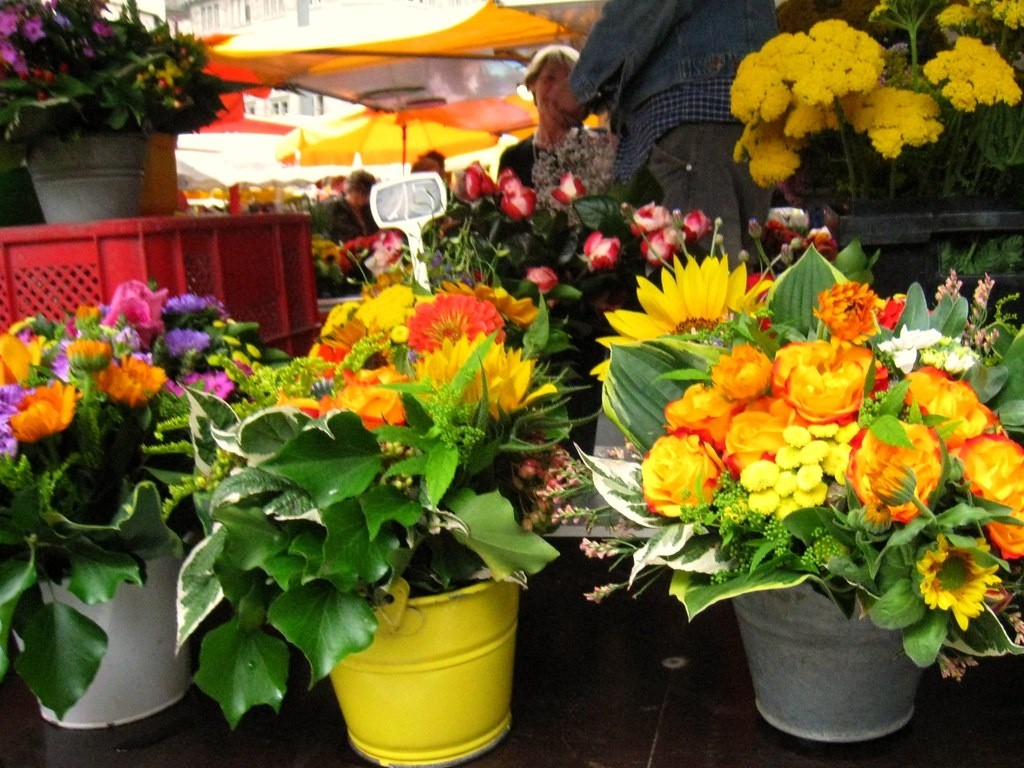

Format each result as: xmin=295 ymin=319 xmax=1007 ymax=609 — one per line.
xmin=0 ymin=214 xmax=321 ymax=357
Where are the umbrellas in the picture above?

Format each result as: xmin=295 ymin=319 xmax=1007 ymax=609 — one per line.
xmin=175 ymin=0 xmax=610 ymax=218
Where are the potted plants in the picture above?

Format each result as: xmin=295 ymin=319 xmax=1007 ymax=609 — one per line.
xmin=934 ymin=235 xmax=1024 ymax=325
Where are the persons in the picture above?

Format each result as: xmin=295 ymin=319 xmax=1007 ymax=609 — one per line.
xmin=316 ymin=151 xmax=472 ymax=286
xmin=566 ymin=1 xmax=781 ymax=270
xmin=497 ymin=45 xmax=618 ymax=268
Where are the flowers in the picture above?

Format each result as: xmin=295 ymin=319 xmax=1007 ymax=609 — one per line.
xmin=152 ymin=287 xmax=604 ymax=729
xmin=0 ymin=280 xmax=289 ymax=722
xmin=0 ymin=0 xmax=259 ymax=170
xmin=542 ymin=248 xmax=1024 ymax=680
xmin=315 ymin=0 xmax=1024 ymax=383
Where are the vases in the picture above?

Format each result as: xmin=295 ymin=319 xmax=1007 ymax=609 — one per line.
xmin=22 ymin=137 xmax=149 ymax=224
xmin=138 ymin=134 xmax=179 ymax=218
xmin=837 ymin=214 xmax=1024 ymax=307
xmin=326 ymin=579 xmax=519 ymax=768
xmin=13 ymin=552 xmax=191 ymax=730
xmin=730 ymin=584 xmax=924 ymax=741
